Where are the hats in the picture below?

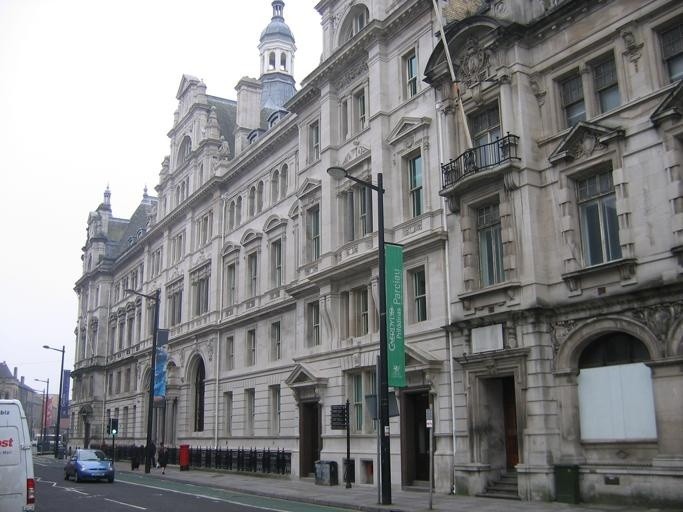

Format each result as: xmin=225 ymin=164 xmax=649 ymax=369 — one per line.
xmin=63 ymin=448 xmax=115 ymax=483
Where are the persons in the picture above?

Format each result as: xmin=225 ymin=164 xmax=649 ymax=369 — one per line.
xmin=99 ymin=440 xmax=169 ymax=475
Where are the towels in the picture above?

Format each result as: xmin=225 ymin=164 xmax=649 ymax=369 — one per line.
xmin=314 ymin=461 xmax=339 ymax=486
xmin=58 ymin=448 xmax=65 ymax=459
xmin=554 ymin=463 xmax=580 ymax=504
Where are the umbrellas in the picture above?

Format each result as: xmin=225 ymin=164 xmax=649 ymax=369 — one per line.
xmin=161 ymin=471 xmax=164 ymax=474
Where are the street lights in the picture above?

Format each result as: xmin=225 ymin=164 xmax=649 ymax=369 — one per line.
xmin=156 ymin=463 xmax=160 ymax=469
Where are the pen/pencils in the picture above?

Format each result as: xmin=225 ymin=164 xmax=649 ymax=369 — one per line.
xmin=0 ymin=399 xmax=36 ymax=512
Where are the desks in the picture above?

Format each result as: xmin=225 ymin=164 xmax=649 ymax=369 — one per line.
xmin=123 ymin=289 xmax=161 ymax=473
xmin=34 ymin=378 xmax=49 ymax=451
xmin=327 ymin=166 xmax=392 ymax=506
xmin=42 ymin=345 xmax=65 ymax=458
